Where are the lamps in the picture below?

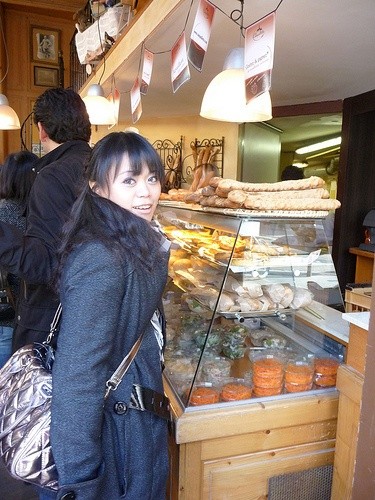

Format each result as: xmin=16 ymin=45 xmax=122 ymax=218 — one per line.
xmin=0 ymin=93 xmax=22 ymax=130
xmin=83 ymin=0 xmax=114 ymax=124
xmin=200 ymin=0 xmax=273 ymax=123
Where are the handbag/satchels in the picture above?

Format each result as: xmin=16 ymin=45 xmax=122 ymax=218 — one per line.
xmin=0 ymin=271 xmax=15 ymax=321
xmin=0 ymin=343 xmax=60 ymax=492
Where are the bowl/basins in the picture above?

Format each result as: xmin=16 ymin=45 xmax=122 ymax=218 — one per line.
xmin=163 ymin=300 xmax=344 ymax=407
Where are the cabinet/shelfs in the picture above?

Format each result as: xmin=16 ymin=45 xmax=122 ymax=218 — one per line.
xmin=153 ymin=202 xmax=350 ymax=500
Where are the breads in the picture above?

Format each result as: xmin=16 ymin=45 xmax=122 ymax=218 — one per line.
xmin=160 ymin=149 xmax=342 ymax=211
xmin=157 ymin=225 xmax=314 ymax=313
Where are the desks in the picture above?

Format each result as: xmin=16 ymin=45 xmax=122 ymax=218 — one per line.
xmin=348 ymin=246 xmax=375 ymax=284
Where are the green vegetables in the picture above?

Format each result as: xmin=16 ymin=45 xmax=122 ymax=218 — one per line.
xmin=178 ymin=311 xmax=246 ymax=360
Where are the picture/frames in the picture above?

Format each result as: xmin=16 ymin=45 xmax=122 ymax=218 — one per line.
xmin=29 ymin=24 xmax=61 ymax=66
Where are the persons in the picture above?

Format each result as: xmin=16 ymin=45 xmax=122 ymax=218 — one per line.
xmin=0 ymin=150 xmax=39 ymax=370
xmin=191 ymin=164 xmax=220 ymax=190
xmin=1 ymin=87 xmax=94 ymax=369
xmin=34 ymin=131 xmax=171 ymax=500
